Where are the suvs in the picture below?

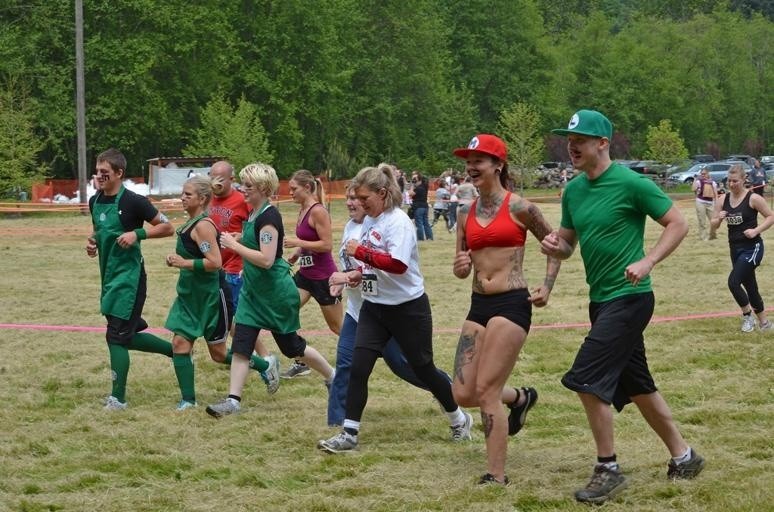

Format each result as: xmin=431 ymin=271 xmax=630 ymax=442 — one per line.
xmin=728 ymin=161 xmax=752 ymax=184
xmin=669 ymin=162 xmax=733 ymax=188
xmin=627 ymin=160 xmax=667 ymax=184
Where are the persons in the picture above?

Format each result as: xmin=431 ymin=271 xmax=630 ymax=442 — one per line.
xmin=429 ymin=180 xmax=451 ymax=228
xmin=208 ymin=161 xmax=272 ymax=360
xmin=205 ymin=164 xmax=335 ymax=418
xmin=452 ymin=134 xmax=561 ymax=490
xmin=748 ymin=160 xmax=768 ymax=196
xmin=401 ymin=172 xmax=410 ymax=203
xmin=717 ymin=182 xmax=726 ymax=195
xmin=459 ymin=177 xmax=465 ymax=184
xmin=281 ymin=170 xmax=345 ymax=382
xmin=710 ymin=165 xmax=773 ymax=333
xmin=451 ymin=175 xmax=478 ymax=233
xmin=691 ymin=169 xmax=717 ymax=241
xmin=446 ymin=176 xmax=464 ymax=228
xmin=187 ymin=169 xmax=195 ymax=179
xmin=409 ymin=174 xmax=433 ymax=241
xmin=318 ymin=163 xmax=474 ymax=455
xmin=560 ymin=167 xmax=567 ymax=183
xmin=328 ymin=182 xmax=426 ymax=431
xmin=165 ymin=175 xmax=281 ymax=413
xmin=393 ymin=169 xmax=403 ymax=192
xmin=540 ymin=109 xmax=688 ymax=495
xmin=86 ymin=149 xmax=175 ymax=411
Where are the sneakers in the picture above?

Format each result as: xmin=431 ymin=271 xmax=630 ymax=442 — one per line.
xmin=175 ymin=398 xmax=200 ymax=410
xmin=259 ymin=355 xmax=281 ymax=395
xmin=279 ymin=359 xmax=312 ymax=379
xmin=476 ymin=472 xmax=510 ymax=488
xmin=759 ymin=318 xmax=773 ymax=332
xmin=507 ymin=386 xmax=538 ymax=437
xmin=103 ymin=394 xmax=129 ymax=410
xmin=666 ymin=449 xmax=707 ymax=481
xmin=449 ymin=410 xmax=475 ymax=444
xmin=205 ymin=399 xmax=236 ymax=420
xmin=574 ymin=462 xmax=629 ymax=504
xmin=740 ymin=314 xmax=757 ymax=333
xmin=315 ymin=432 xmax=361 ymax=455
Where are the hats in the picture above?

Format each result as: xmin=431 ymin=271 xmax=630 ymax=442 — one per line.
xmin=453 ymin=133 xmax=507 ymax=161
xmin=550 ymin=110 xmax=613 ymax=142
